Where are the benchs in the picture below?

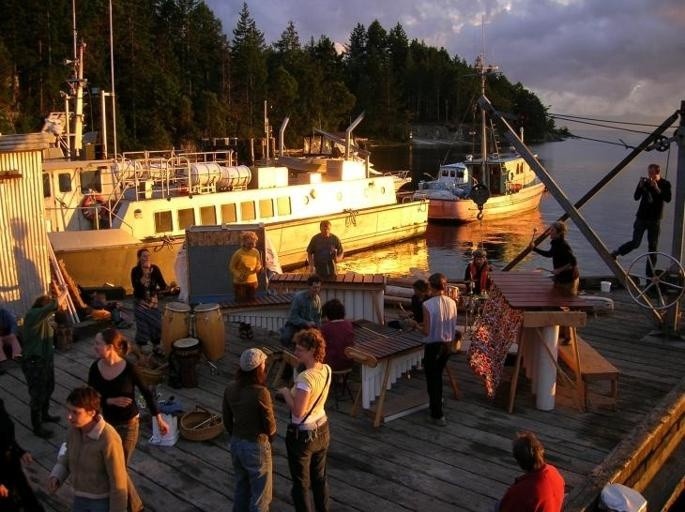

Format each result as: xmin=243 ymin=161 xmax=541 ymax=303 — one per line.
xmin=559 ymin=335 xmax=624 ymax=413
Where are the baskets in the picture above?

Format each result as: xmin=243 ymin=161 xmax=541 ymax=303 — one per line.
xmin=180 ymin=404 xmax=225 ymax=442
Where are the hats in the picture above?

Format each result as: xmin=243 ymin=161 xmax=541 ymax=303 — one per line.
xmin=239 ymin=346 xmax=267 ymax=371
xmin=472 ymin=249 xmax=487 ymax=257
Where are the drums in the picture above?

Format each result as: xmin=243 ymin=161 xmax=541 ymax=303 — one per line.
xmin=194 ymin=303 xmax=226 ymax=362
xmin=161 ymin=302 xmax=190 ymax=354
xmin=172 ymin=337 xmax=202 ymax=367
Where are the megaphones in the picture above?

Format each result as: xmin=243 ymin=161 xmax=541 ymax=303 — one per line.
xmin=64 ymin=59 xmax=72 ymax=65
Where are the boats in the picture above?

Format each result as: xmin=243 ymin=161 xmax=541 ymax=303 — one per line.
xmin=420 ymin=209 xmax=549 ymax=274
xmin=410 ymin=50 xmax=550 ymax=225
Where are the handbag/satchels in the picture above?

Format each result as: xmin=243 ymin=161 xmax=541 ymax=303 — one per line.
xmin=286 ymin=423 xmax=299 ymax=443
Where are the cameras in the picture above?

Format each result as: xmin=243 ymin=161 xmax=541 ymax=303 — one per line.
xmin=642 ymin=178 xmax=650 ymax=183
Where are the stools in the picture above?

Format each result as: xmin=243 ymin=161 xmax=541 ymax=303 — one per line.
xmin=330 ymin=368 xmax=355 ymax=410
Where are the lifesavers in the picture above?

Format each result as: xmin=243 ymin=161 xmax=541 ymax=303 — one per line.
xmin=82 ymin=194 xmax=110 ymax=222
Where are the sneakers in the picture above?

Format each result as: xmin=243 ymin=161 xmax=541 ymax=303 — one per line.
xmin=40 ymin=412 xmax=62 ymax=424
xmin=426 ymin=415 xmax=448 ymax=427
xmin=33 ymin=426 xmax=54 ymax=438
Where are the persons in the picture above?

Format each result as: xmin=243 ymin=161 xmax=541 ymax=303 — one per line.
xmin=281 ymin=277 xmax=323 ymax=346
xmin=596 ymin=483 xmax=647 ymax=512
xmin=228 ymin=231 xmax=263 ymax=339
xmin=281 ymin=330 xmax=333 ymax=512
xmin=464 ymin=249 xmax=496 ymax=295
xmin=0 ymin=296 xmax=169 ymax=512
xmin=409 ymin=273 xmax=457 ymax=426
xmin=388 ymin=281 xmax=431 ymax=333
xmin=530 ymin=220 xmax=579 ymax=344
xmin=306 ymin=220 xmax=344 ymax=276
xmin=612 ymin=164 xmax=672 ymax=284
xmin=130 ymin=249 xmax=171 ymax=358
xmin=496 ymin=431 xmax=566 ymax=512
xmin=222 ymin=347 xmax=278 ymax=512
xmin=317 ymin=298 xmax=355 ymax=374
